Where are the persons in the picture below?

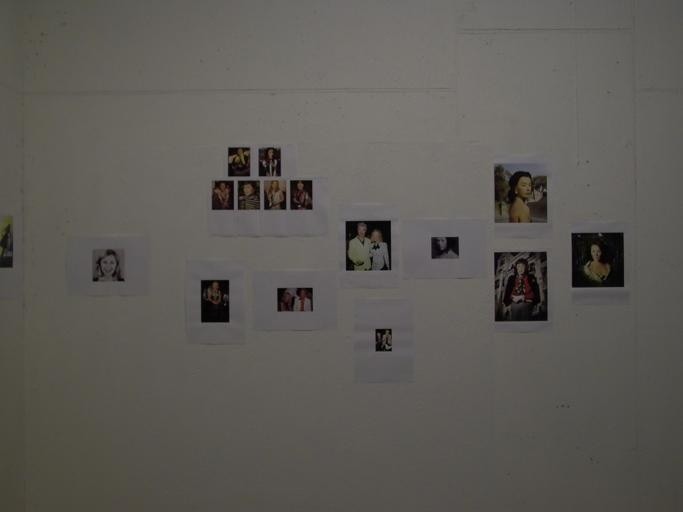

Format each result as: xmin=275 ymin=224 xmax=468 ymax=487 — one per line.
xmin=369 ymin=229 xmax=391 ymax=271
xmin=92 ymin=248 xmax=123 ymax=282
xmin=505 ymin=172 xmax=535 ymax=223
xmin=375 ymin=329 xmax=392 ymax=351
xmin=578 ymin=241 xmax=612 ymax=284
xmin=503 ymin=258 xmax=541 ymax=321
xmin=213 ymin=147 xmax=312 ymax=210
xmin=345 ymin=221 xmax=371 ymax=271
xmin=207 ymin=282 xmax=222 ymax=319
xmin=436 ymin=237 xmax=459 ymax=259
xmin=292 ymin=289 xmax=313 ymax=312
xmin=279 ymin=292 xmax=292 ymax=311
